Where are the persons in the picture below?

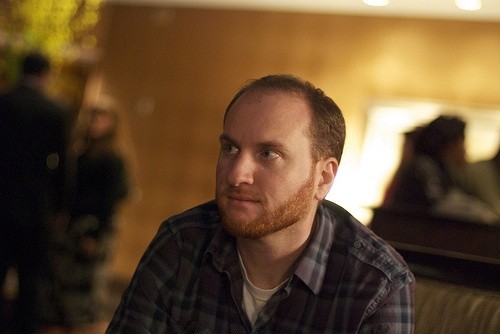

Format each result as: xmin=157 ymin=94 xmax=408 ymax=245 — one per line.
xmin=103 ymin=71 xmax=419 ymax=334
xmin=379 ymin=112 xmax=500 ymax=221
xmin=0 ymin=42 xmax=128 ymax=333
